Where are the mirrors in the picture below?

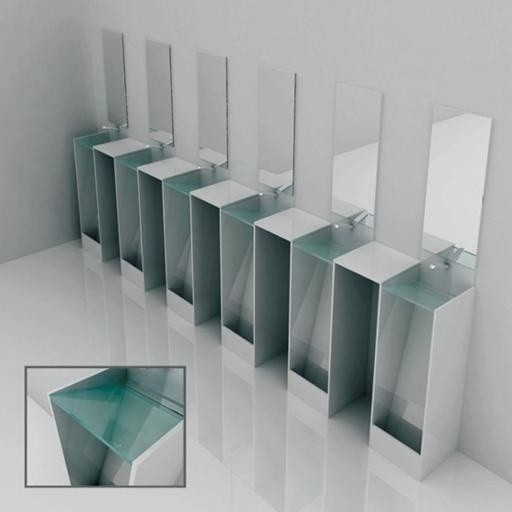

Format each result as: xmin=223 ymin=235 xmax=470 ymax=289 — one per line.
xmin=145 ymin=39 xmax=174 ymax=146
xmin=101 ymin=30 xmax=127 ymax=128
xmin=196 ymin=51 xmax=228 ymax=167
xmin=421 ymin=105 xmax=491 ymax=269
xmin=258 ymin=68 xmax=294 ymax=192
xmin=331 ymin=84 xmax=382 ymax=228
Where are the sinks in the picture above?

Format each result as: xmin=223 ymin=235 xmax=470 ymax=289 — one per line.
xmin=220 ymin=162 xmax=228 ymax=169
xmin=438 ymin=245 xmax=476 ymax=270
xmin=381 ymin=280 xmax=457 ymax=311
xmin=117 ymin=155 xmax=155 ymax=171
xmin=76 ymin=138 xmax=109 ymax=149
xmin=49 ymin=384 xmax=184 ymax=464
xmin=283 ymin=185 xmax=292 ymax=194
xmin=356 ymin=209 xmax=375 ymax=228
xmin=220 ymin=207 xmax=270 ymax=226
xmin=163 ymin=179 xmax=202 ymax=196
xmin=292 ymin=240 xmax=349 ymax=264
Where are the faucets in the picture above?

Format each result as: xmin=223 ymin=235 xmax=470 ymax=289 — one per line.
xmin=450 ymin=247 xmax=463 ymax=260
xmin=258 ymin=191 xmax=277 ymax=200
xmin=333 ymin=223 xmax=356 ymax=234
xmin=199 ymin=166 xmax=214 ymax=172
xmin=102 ymin=126 xmax=119 ymax=132
xmin=146 ymin=145 xmax=163 ymax=151
xmin=430 ymin=262 xmax=450 ymax=274
